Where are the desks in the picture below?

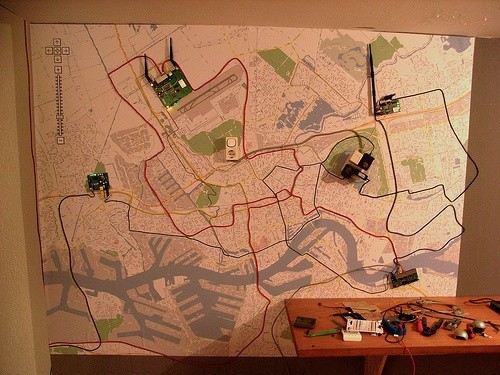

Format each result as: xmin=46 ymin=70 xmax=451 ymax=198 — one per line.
xmin=284 ymin=296 xmax=500 ymax=375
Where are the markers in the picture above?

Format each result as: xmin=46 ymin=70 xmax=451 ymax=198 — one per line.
xmin=468 ymin=324 xmax=476 ymax=338
xmin=418 ymin=318 xmax=423 ymax=332
